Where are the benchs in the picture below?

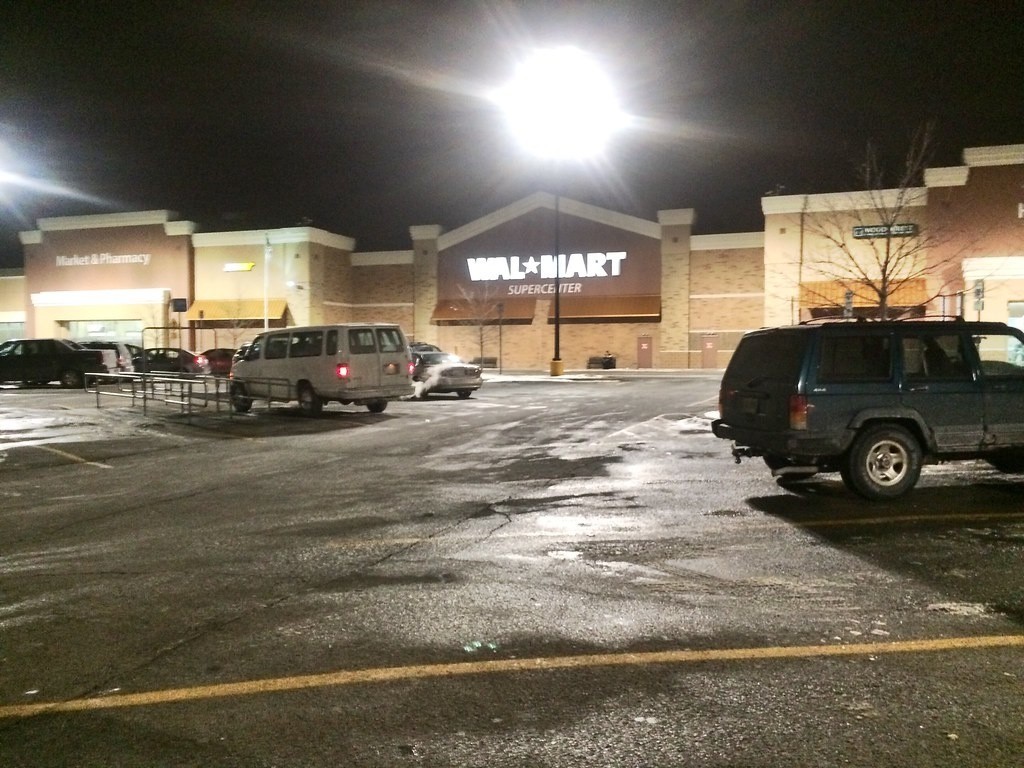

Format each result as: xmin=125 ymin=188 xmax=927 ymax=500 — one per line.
xmin=470 ymin=357 xmax=498 ymax=368
xmin=587 ymin=357 xmax=607 ymax=369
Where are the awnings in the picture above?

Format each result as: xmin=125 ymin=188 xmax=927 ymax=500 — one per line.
xmin=799 ymin=278 xmax=926 ymax=308
xmin=186 ymin=299 xmax=287 ymax=321
xmin=547 ymin=294 xmax=661 ymax=318
xmin=430 ymin=296 xmax=536 ymax=321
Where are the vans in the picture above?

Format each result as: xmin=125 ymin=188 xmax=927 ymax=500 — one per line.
xmin=230 ymin=323 xmax=411 ymax=419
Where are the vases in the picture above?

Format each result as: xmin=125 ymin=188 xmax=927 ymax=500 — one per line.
xmin=602 ymin=358 xmax=616 ymax=368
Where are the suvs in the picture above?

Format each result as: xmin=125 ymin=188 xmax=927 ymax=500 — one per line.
xmin=385 ymin=341 xmax=441 ymax=354
xmin=232 ymin=343 xmax=260 ymax=367
xmin=712 ymin=311 xmax=1024 ymax=502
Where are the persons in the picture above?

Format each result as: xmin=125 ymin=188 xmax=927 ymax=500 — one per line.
xmin=602 ymin=351 xmax=613 ymax=369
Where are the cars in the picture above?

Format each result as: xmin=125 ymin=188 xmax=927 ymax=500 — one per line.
xmin=0 ymin=339 xmax=118 ymax=390
xmin=409 ymin=350 xmax=483 ymax=398
xmin=76 ymin=340 xmax=145 ymax=382
xmin=201 ymin=348 xmax=237 ymax=374
xmin=131 ymin=347 xmax=203 ymax=375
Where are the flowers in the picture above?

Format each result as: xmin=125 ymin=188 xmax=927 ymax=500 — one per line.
xmin=604 ymin=351 xmax=613 ymax=357
